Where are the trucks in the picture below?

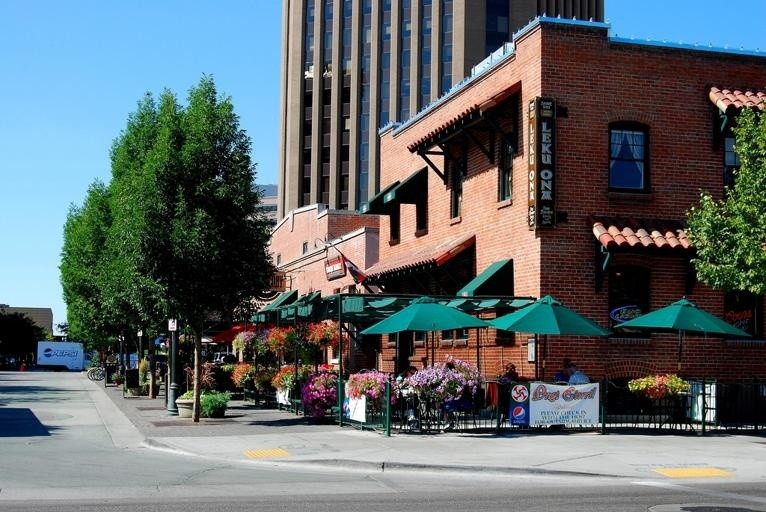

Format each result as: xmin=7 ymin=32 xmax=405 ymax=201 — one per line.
xmin=37 ymin=339 xmax=93 ymax=372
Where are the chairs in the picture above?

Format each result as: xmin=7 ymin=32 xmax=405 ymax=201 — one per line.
xmin=393 ymin=374 xmax=480 ymax=433
xmin=491 ymin=380 xmax=601 ymax=431
xmin=624 ymin=385 xmax=695 ymax=433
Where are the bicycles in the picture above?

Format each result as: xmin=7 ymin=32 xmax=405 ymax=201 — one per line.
xmin=85 ymin=360 xmax=121 ymax=381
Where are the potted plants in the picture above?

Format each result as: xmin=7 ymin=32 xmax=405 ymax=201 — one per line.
xmin=111 ymin=356 xmax=231 ymax=417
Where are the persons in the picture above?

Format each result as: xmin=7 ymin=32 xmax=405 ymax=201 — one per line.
xmin=567 ymin=364 xmax=590 ymax=383
xmin=499 ymin=363 xmax=518 ymax=384
xmin=554 ymin=358 xmax=572 ymax=382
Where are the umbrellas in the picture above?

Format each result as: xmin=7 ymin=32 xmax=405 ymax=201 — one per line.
xmin=485 ymin=295 xmax=613 ymax=381
xmin=613 ymin=296 xmax=752 ymax=377
xmin=359 ymin=295 xmax=496 ymax=367
